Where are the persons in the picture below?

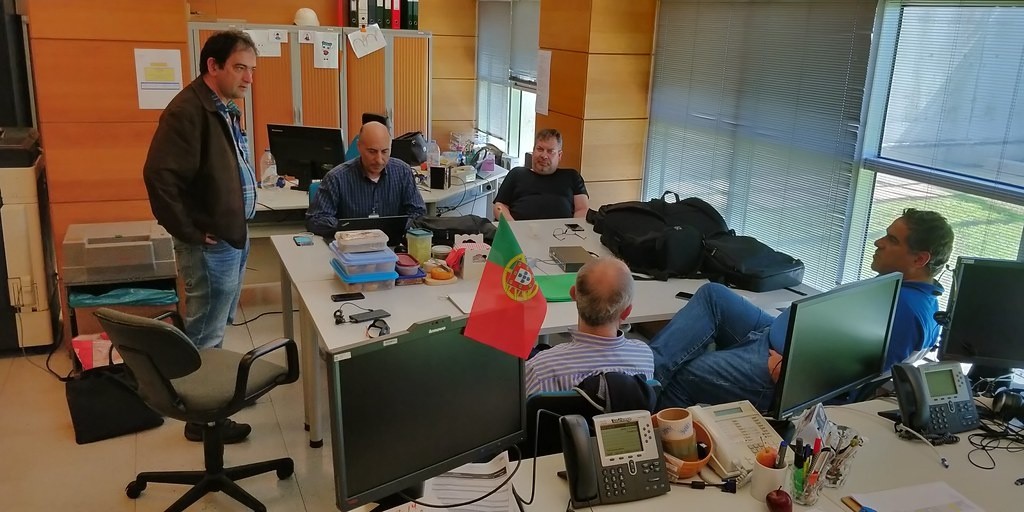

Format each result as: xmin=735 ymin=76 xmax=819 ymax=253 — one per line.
xmin=143 ymin=29 xmax=258 ymax=445
xmin=524 ymin=255 xmax=654 ymax=398
xmin=304 ymin=121 xmax=427 ymax=237
xmin=493 ymin=128 xmax=589 ymax=221
xmin=646 ymin=207 xmax=954 ymax=414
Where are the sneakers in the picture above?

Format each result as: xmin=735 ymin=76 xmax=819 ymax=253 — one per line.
xmin=184 ymin=417 xmax=251 ymax=444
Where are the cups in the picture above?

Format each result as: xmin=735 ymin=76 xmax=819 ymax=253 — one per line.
xmin=407 ymin=228 xmax=433 ymax=269
xmin=789 ymin=455 xmax=855 ymax=506
xmin=751 ymin=460 xmax=788 ymax=502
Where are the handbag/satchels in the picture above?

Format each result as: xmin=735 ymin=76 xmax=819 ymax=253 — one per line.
xmin=699 ymin=228 xmax=805 ymax=292
xmin=72 ymin=327 xmax=123 ymax=370
xmin=593 ymin=190 xmax=736 ymax=281
xmin=406 ymin=213 xmax=498 ymax=246
xmin=390 ymin=128 xmax=429 ymax=168
xmin=65 ymin=339 xmax=165 ymax=445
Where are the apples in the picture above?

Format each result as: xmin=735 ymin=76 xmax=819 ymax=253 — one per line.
xmin=756 ymin=447 xmax=778 ymax=467
xmin=766 ymin=486 xmax=793 ymax=512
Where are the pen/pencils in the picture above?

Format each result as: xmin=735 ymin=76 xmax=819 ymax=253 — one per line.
xmin=827 ymin=432 xmax=868 ymax=485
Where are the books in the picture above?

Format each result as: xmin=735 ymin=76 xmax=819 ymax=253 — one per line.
xmin=841 ymin=481 xmax=988 ymax=512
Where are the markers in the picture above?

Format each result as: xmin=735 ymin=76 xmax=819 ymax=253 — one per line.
xmin=441 ymin=264 xmax=451 ymax=272
xmin=774 ymin=441 xmax=788 ymax=469
xmin=721 ymin=478 xmax=737 ymax=482
xmin=794 ymin=438 xmax=822 ymax=499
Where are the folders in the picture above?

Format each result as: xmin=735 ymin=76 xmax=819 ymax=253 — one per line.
xmin=339 ymin=0 xmax=418 ymax=30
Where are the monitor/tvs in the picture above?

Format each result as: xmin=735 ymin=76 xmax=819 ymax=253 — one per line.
xmin=773 ymin=271 xmax=903 ymax=421
xmin=325 ymin=311 xmax=527 ymax=512
xmin=267 ymin=124 xmax=347 ymax=191
xmin=938 ymin=255 xmax=1024 ymax=398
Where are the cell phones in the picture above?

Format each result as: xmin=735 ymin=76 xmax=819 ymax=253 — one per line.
xmin=565 ymin=224 xmax=584 ymax=231
xmin=331 ymin=293 xmax=365 ymax=302
xmin=349 ymin=311 xmax=391 ymax=321
xmin=294 ymin=236 xmax=314 ymax=246
xmin=675 ymin=291 xmax=694 ymax=301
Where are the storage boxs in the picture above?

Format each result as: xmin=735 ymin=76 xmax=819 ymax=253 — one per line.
xmin=71 ymin=286 xmax=177 ymax=336
xmin=329 ymin=228 xmax=399 ymax=292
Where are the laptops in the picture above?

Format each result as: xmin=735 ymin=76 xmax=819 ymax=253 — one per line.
xmin=324 ymin=215 xmax=411 ymax=246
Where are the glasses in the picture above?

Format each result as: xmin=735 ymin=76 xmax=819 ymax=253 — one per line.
xmin=365 ymin=318 xmax=390 ymax=338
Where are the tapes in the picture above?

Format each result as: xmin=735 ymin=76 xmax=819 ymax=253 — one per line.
xmin=662 ymin=428 xmax=698 ymax=462
xmin=657 ymin=408 xmax=693 ymax=440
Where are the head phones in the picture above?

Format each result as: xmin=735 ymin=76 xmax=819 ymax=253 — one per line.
xmin=991 ymin=388 xmax=1024 ymax=426
xmin=932 ymin=269 xmax=957 ymax=324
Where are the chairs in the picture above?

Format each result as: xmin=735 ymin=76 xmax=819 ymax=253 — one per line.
xmin=92 ymin=308 xmax=300 ymax=512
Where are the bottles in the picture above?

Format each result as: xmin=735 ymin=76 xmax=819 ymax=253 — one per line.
xmin=427 ymin=140 xmax=441 ymax=187
xmin=260 ymin=149 xmax=277 ymax=190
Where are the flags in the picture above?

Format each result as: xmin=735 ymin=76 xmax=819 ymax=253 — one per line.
xmin=464 ymin=210 xmax=547 ymax=360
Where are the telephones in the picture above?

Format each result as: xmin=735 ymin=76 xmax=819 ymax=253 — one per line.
xmin=558 ymin=409 xmax=671 ymax=509
xmin=891 ymin=360 xmax=980 ymax=439
xmin=687 ymin=400 xmax=795 ymax=479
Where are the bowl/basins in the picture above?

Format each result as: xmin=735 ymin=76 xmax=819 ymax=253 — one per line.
xmin=651 ymin=414 xmax=713 ymax=479
xmin=432 ymin=245 xmax=451 ymax=259
xmin=329 ymin=229 xmax=426 ymax=291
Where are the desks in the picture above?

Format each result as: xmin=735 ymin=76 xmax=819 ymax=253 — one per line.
xmin=269 ymin=217 xmax=818 ymax=450
xmin=507 ymin=393 xmax=1024 ymax=512
xmin=242 ymin=156 xmax=506 ymax=245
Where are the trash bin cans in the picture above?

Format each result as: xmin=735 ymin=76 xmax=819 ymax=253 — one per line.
xmin=69 ymin=287 xmax=176 ymax=335
